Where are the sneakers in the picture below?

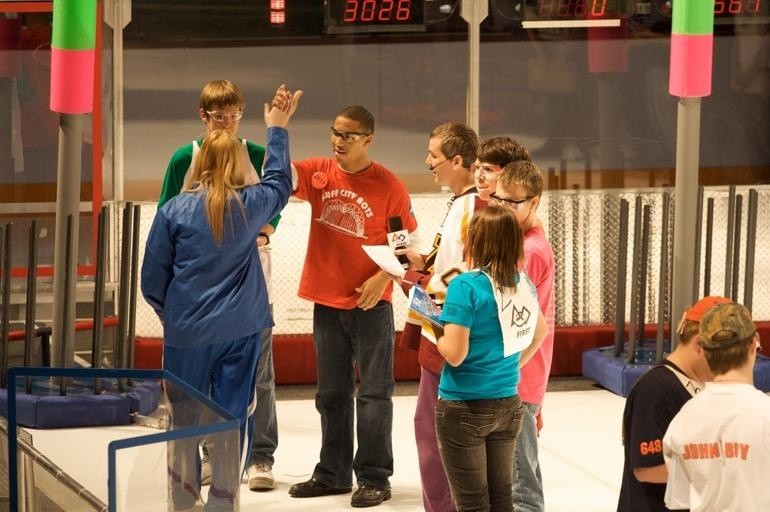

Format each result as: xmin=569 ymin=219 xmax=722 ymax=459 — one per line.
xmin=351 ymin=486 xmax=391 ymax=507
xmin=201 ymin=457 xmax=212 ymax=486
xmin=289 ymin=476 xmax=352 ymax=497
xmin=248 ymin=464 xmax=274 ymax=490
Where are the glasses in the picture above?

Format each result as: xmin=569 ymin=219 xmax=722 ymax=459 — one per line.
xmin=206 ymin=110 xmax=242 ymax=121
xmin=331 ymin=127 xmax=368 ymax=142
xmin=488 ymin=191 xmax=534 ymax=210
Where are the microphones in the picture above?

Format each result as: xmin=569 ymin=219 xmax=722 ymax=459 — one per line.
xmin=388 ymin=216 xmax=410 ymax=270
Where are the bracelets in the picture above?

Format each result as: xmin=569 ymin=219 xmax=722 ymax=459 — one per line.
xmin=258 ymin=230 xmax=272 ymax=245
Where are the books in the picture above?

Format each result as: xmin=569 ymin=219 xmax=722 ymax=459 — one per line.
xmin=405 ymin=286 xmax=448 ymax=339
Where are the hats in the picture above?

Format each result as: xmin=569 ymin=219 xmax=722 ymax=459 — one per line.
xmin=685 ymin=297 xmax=733 ymax=323
xmin=698 ymin=303 xmax=757 ymax=351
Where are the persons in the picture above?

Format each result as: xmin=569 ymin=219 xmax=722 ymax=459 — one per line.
xmin=661 ymin=303 xmax=770 ymax=512
xmin=487 ymin=158 xmax=557 ymax=512
xmin=264 ymin=84 xmax=419 ymax=507
xmin=614 ymin=296 xmax=737 ymax=512
xmin=140 ymin=90 xmax=296 ymax=511
xmin=475 ymin=137 xmax=543 ymax=438
xmin=426 ymin=206 xmax=550 ymax=511
xmin=157 ymin=80 xmax=280 ymax=490
xmin=386 ymin=120 xmax=478 ymax=511
